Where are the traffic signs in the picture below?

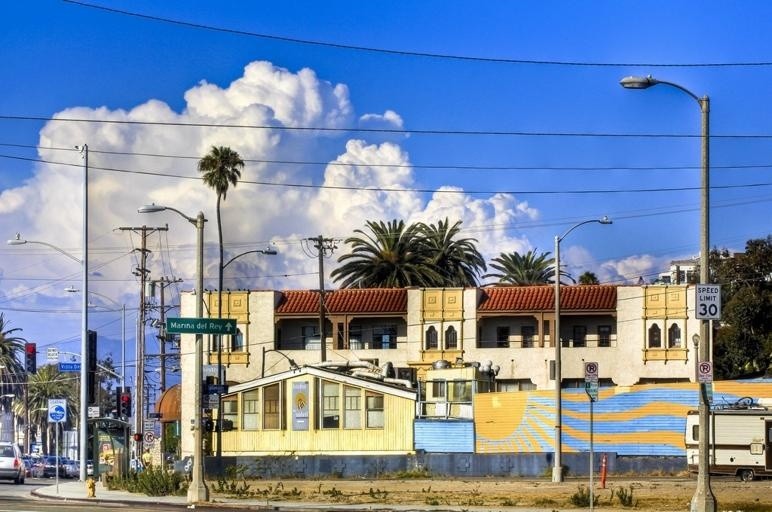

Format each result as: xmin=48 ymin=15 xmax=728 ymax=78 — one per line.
xmin=696 ymin=283 xmax=721 ymax=320
xmin=167 ymin=318 xmax=238 ymax=335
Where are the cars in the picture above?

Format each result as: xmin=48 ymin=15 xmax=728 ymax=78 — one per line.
xmin=0 ymin=441 xmax=93 ymax=485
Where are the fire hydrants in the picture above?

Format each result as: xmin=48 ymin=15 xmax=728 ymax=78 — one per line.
xmin=86 ymin=475 xmax=96 ymax=497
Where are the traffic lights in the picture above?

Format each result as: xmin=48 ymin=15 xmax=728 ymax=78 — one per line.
xmin=120 ymin=393 xmax=131 ymax=417
xmin=25 ymin=342 xmax=37 ymax=376
xmin=134 ymin=433 xmax=142 ymax=441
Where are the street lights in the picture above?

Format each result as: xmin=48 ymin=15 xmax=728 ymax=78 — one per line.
xmin=619 ymin=74 xmax=717 ymax=512
xmin=6 ymin=144 xmax=89 ymax=481
xmin=64 ymin=285 xmax=125 ymax=393
xmin=138 ymin=203 xmax=209 ymax=503
xmin=223 ymin=247 xmax=277 ymax=269
xmin=692 ymin=334 xmax=700 ymax=382
xmin=552 ymin=216 xmax=612 ymax=483
